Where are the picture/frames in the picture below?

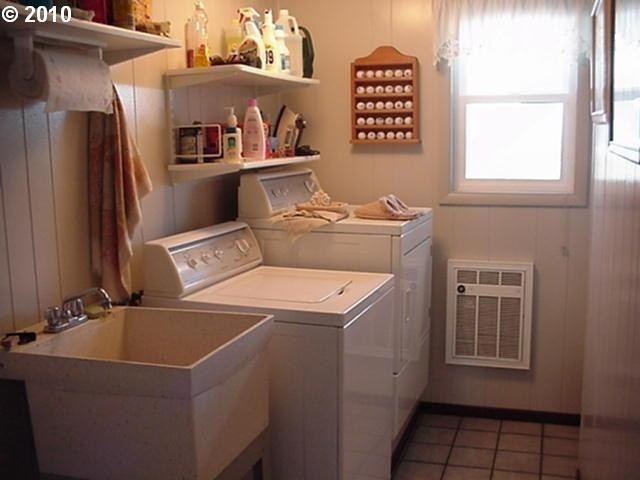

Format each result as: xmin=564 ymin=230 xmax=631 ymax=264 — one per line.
xmin=586 ymin=0 xmax=611 ymax=126
xmin=607 ymin=0 xmax=640 ymax=165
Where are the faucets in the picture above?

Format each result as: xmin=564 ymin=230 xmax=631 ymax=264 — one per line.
xmin=42 ymin=286 xmax=114 ymax=335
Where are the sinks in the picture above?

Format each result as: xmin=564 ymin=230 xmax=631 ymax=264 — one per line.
xmin=3 ymin=307 xmax=274 ymax=479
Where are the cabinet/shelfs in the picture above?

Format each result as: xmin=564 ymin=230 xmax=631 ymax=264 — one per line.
xmin=349 ymin=46 xmax=421 ymax=145
xmin=163 ymin=65 xmax=321 ymax=187
xmin=0 ymin=1 xmax=184 ymax=101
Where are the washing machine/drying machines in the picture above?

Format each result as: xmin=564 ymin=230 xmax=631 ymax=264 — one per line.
xmin=143 ymin=221 xmax=395 ymax=480
xmin=238 ymin=164 xmax=434 ymax=439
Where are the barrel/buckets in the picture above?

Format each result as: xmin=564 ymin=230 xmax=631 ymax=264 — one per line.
xmin=272 ymin=9 xmax=306 ymax=81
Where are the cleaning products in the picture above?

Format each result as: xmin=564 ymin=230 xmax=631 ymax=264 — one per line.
xmin=262 ymin=11 xmax=281 ymax=75
xmin=242 ymin=99 xmax=265 ymax=160
xmin=274 ymin=24 xmax=290 ymax=75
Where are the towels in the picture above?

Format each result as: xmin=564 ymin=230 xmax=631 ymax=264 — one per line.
xmin=88 ymin=81 xmax=154 ymax=305
xmin=270 ymin=200 xmax=350 ymax=243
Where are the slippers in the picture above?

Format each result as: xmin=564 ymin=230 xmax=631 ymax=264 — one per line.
xmin=388 ymin=194 xmax=425 ymax=215
xmin=353 ymin=194 xmax=419 ymax=220
